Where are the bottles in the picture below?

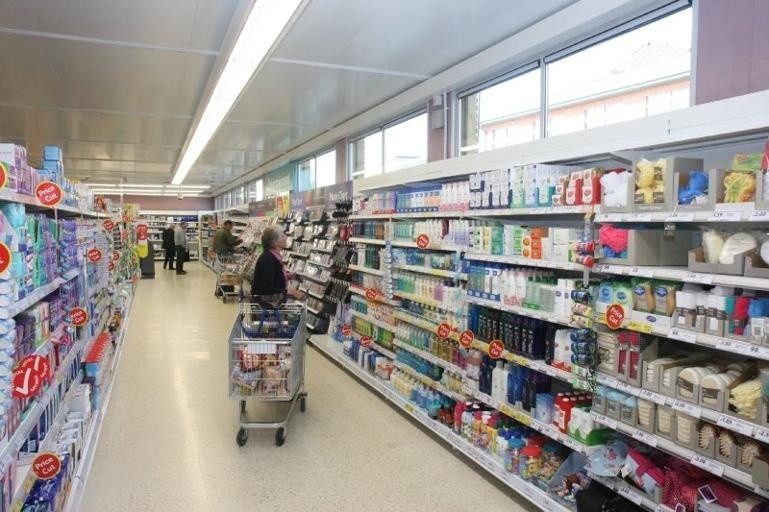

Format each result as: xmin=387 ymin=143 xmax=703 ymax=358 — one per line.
xmin=349 ymin=315 xmax=394 ymax=351
xmin=350 ymin=270 xmax=381 ymax=294
xmin=393 ymin=347 xmax=462 ymax=395
xmin=505 ymin=433 xmax=566 ymax=482
xmin=466 ymin=261 xmax=485 ymax=294
xmin=454 ymin=398 xmax=533 ymax=462
xmin=354 ymin=243 xmax=386 ymax=272
xmin=391 ymin=269 xmax=467 ymax=333
xmin=351 ymin=220 xmax=385 ymax=240
xmin=389 ymin=369 xmax=453 ymax=427
xmin=396 ymin=191 xmax=440 ymax=212
xmin=444 ymin=387 xmax=454 ymax=393
xmin=552 ymin=389 xmax=610 ymax=447
xmin=483 ymin=262 xmax=508 ymax=296
xmin=616 ymin=331 xmax=642 ymax=379
xmin=500 ymin=267 xmax=554 ymax=306
xmin=522 ymin=274 xmax=558 ymax=313
xmin=390 ymin=219 xmax=467 ymax=272
xmin=497 ymin=311 xmax=568 ymax=366
xmin=437 ymin=180 xmax=469 ymax=212
xmin=673 ymin=305 xmax=727 ymax=338
xmin=467 ymin=219 xmax=504 ymax=257
xmin=599 ymin=280 xmax=632 ymax=307
xmin=467 ymin=346 xmax=552 ymax=425
xmin=349 ymin=292 xmax=369 ymax=316
xmin=633 ymin=279 xmax=679 ymax=317
xmin=467 ymin=306 xmax=500 ymax=343
xmin=554 ymin=277 xmax=603 ymax=317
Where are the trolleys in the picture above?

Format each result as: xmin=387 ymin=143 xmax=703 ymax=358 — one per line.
xmin=228 ymin=295 xmax=310 ymax=447
xmin=213 ymin=247 xmax=245 ymax=303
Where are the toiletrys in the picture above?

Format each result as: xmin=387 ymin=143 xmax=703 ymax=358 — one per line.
xmin=401 ymin=298 xmax=467 ymax=334
xmin=469 ymin=220 xmax=503 ymax=254
xmin=352 ymin=272 xmax=383 ymax=293
xmin=466 ymin=343 xmax=551 ymax=416
xmin=467 ymin=264 xmax=573 ymax=316
xmin=455 ymin=397 xmax=531 ymax=457
xmin=350 ymin=296 xmax=395 ymax=326
xmin=350 ymin=244 xmax=387 ymax=268
xmin=352 ymin=220 xmax=468 ymax=247
xmin=394 ymin=274 xmax=466 ymax=307
xmin=372 ymin=181 xmax=468 ymax=211
xmin=390 ymin=367 xmax=453 ymax=426
xmin=399 ymin=323 xmax=466 ymax=371
xmin=553 ymin=389 xmax=609 ymax=441
xmin=391 ymin=248 xmax=458 ymax=272
xmin=472 ymin=307 xmax=574 ymax=370
xmin=353 ymin=314 xmax=392 ymax=350
xmin=396 ymin=348 xmax=462 ymax=394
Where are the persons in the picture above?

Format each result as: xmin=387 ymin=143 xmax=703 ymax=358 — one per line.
xmin=162 ymin=221 xmax=177 ymax=272
xmin=174 ymin=220 xmax=189 ymax=275
xmin=249 ymin=227 xmax=306 ymax=332
xmin=212 ymin=220 xmax=244 ymax=299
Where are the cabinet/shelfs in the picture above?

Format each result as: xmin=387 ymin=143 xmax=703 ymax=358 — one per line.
xmin=140 ymin=213 xmax=199 ymax=261
xmin=454 ymin=205 xmax=593 ymax=511
xmin=587 ymin=211 xmax=769 ymax=512
xmin=199 ymin=207 xmax=348 ymax=348
xmin=309 ymin=211 xmax=452 ymax=443
xmin=0 ymin=180 xmax=136 ymax=511
